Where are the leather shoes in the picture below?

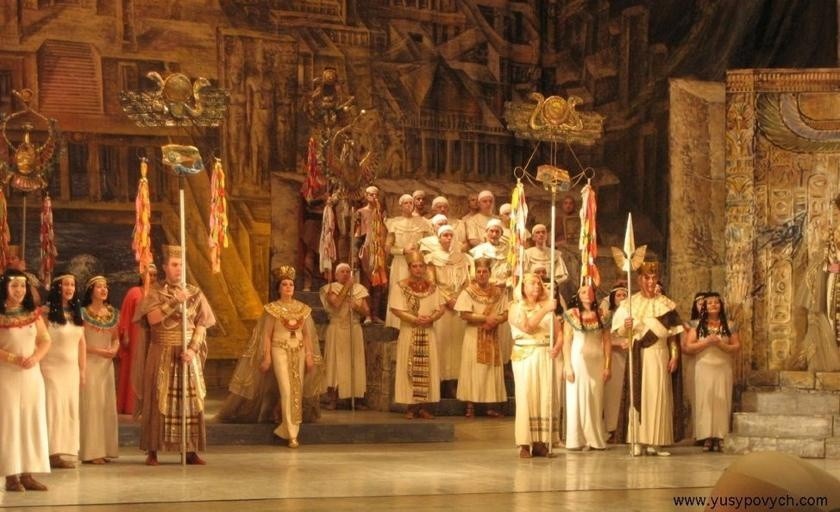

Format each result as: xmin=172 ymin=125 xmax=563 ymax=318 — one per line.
xmin=646 ymin=444 xmax=672 ymax=458
xmin=288 ymin=439 xmax=299 ymax=448
xmin=629 ymin=445 xmax=643 ymax=457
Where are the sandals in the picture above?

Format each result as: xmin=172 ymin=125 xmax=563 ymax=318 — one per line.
xmin=180 ymin=452 xmax=206 ymax=467
xmin=519 ymin=445 xmax=530 ymax=458
xmin=352 ymin=402 xmax=369 ymax=413
xmin=19 ymin=472 xmax=48 ymax=493
xmin=702 ymin=439 xmax=715 ymax=453
xmin=417 ymin=409 xmax=435 ymax=420
xmin=532 ymin=442 xmax=556 ymax=458
xmin=5 ymin=476 xmax=25 ymax=493
xmin=403 ymin=408 xmax=416 ymax=421
xmin=50 ymin=455 xmax=76 ymax=470
xmin=326 ymin=400 xmax=339 ymax=412
xmin=717 ymin=440 xmax=725 ymax=451
xmin=83 ymin=459 xmax=107 ymax=465
xmin=144 ymin=452 xmax=159 ymax=466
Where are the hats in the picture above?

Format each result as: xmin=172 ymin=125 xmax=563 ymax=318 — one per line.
xmin=480 ymin=408 xmax=505 ymax=419
xmin=465 ymin=407 xmax=475 ymax=418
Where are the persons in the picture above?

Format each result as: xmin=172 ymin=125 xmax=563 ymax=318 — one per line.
xmin=428 ymin=226 xmax=469 ymax=395
xmin=454 ymin=259 xmax=508 ymax=418
xmin=386 ymin=195 xmax=435 ymax=258
xmin=463 ymin=191 xmax=506 ymax=252
xmin=684 ymin=291 xmax=741 ymax=452
xmin=120 ymin=265 xmax=156 ymax=414
xmin=319 ymin=263 xmax=368 ymax=410
xmin=599 ymin=286 xmax=640 ymax=443
xmin=411 ymin=191 xmax=427 ymax=216
xmin=39 ymin=272 xmax=87 ymax=469
xmin=81 ymin=276 xmax=120 ymax=464
xmin=522 ymin=225 xmax=569 ymax=287
xmin=261 ymin=268 xmax=317 ymax=449
xmin=134 ymin=246 xmax=215 ymax=464
xmin=498 ymin=203 xmax=516 ymax=230
xmin=551 ymin=195 xmax=581 ymax=245
xmin=471 ymin=220 xmax=513 ymax=367
xmin=610 ymin=261 xmax=678 ymax=456
xmin=678 ymin=292 xmax=706 ymax=438
xmin=654 ymin=282 xmax=665 ymax=296
xmin=355 ymin=187 xmax=386 ymax=326
xmin=429 ymin=197 xmax=458 ymax=225
xmin=389 ymin=251 xmax=445 ymax=422
xmin=0 ymin=269 xmax=52 ymax=493
xmin=563 ymin=285 xmax=610 ymax=451
xmin=511 ymin=275 xmax=563 ymax=457
